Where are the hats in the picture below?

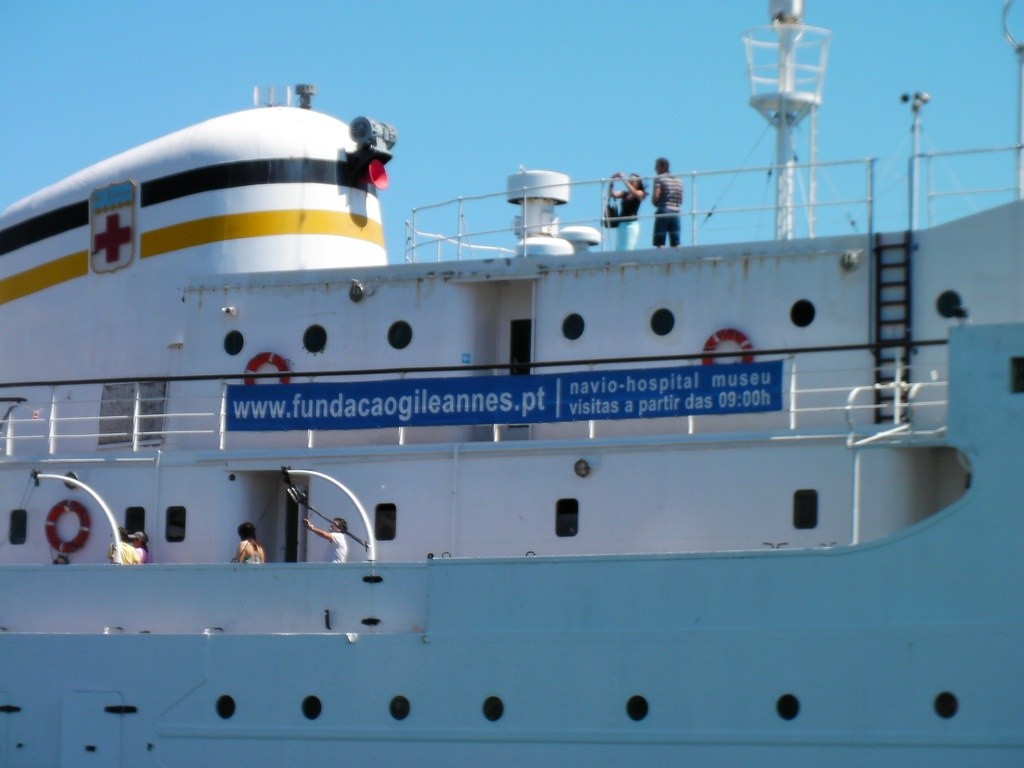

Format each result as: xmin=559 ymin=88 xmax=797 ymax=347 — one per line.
xmin=128 ymin=531 xmax=148 ymax=543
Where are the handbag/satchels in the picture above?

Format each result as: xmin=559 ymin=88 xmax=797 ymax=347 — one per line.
xmin=601 ymin=196 xmax=619 ymax=228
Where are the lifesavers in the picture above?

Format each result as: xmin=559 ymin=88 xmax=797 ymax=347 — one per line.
xmin=244 ymin=352 xmax=290 ymax=385
xmin=701 ymin=328 xmax=753 ymax=364
xmin=44 ymin=499 xmax=91 ymax=553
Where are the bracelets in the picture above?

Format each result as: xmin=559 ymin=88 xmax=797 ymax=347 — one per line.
xmin=309 ymin=524 xmax=314 ymax=531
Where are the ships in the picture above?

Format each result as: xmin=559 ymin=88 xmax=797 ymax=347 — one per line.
xmin=0 ymin=0 xmax=1023 ymax=768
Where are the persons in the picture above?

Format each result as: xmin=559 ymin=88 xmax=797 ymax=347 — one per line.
xmin=653 ymin=158 xmax=682 ymax=247
xmin=608 ymin=173 xmax=648 ymax=251
xmin=236 ymin=522 xmax=264 ymax=564
xmin=109 ymin=527 xmax=149 ymax=564
xmin=304 ymin=518 xmax=348 ymax=563
